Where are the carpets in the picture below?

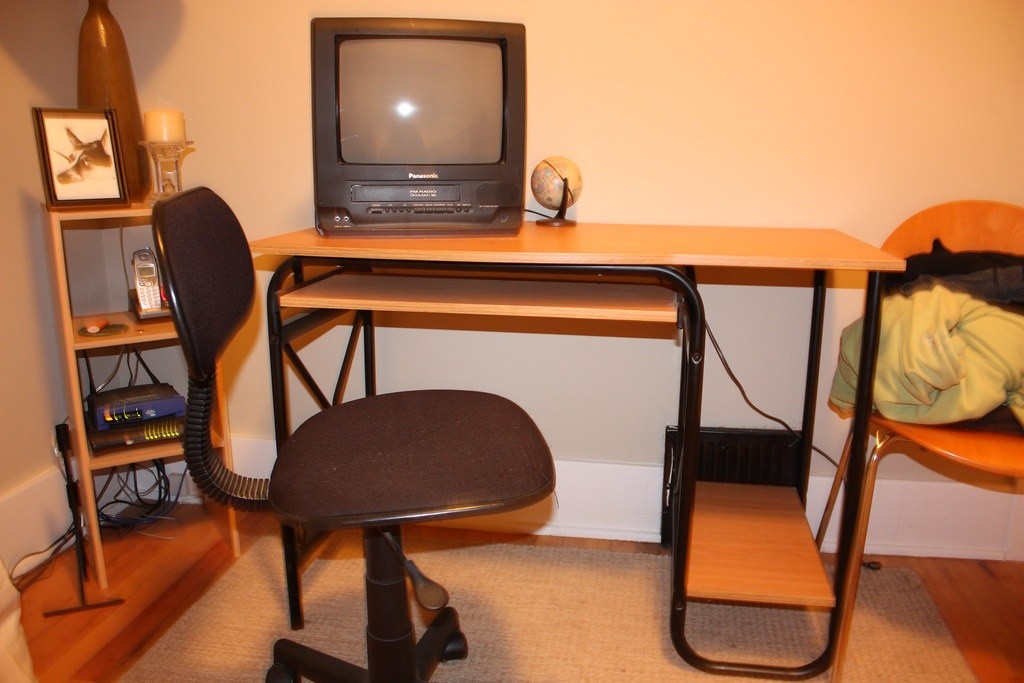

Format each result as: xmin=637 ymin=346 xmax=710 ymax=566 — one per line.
xmin=115 ymin=534 xmax=976 ymax=683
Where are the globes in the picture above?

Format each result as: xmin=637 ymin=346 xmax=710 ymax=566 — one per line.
xmin=531 ymin=156 xmax=582 ymax=226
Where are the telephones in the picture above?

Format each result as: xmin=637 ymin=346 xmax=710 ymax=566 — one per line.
xmin=128 ymin=246 xmax=173 ymax=320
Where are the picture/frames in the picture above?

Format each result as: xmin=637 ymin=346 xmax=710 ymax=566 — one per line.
xmin=32 ymin=107 xmax=131 ymax=213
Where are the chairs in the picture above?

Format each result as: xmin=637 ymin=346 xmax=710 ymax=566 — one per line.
xmin=813 ymin=200 xmax=1024 ymax=683
xmin=159 ymin=189 xmax=557 ymax=683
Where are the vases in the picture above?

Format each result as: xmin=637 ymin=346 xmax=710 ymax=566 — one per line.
xmin=78 ymin=0 xmax=154 ymax=200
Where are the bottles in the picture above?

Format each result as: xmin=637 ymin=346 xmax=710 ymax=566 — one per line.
xmin=78 ymin=1 xmax=153 ymax=202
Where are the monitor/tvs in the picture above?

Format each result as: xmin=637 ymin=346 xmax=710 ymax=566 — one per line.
xmin=309 ymin=16 xmax=527 ymax=238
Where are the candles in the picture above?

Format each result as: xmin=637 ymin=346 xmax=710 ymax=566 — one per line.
xmin=142 ymin=107 xmax=187 ymax=143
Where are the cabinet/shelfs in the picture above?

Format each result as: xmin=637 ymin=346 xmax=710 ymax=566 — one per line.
xmin=248 ymin=220 xmax=908 ymax=680
xmin=39 ymin=202 xmax=240 ymax=590
